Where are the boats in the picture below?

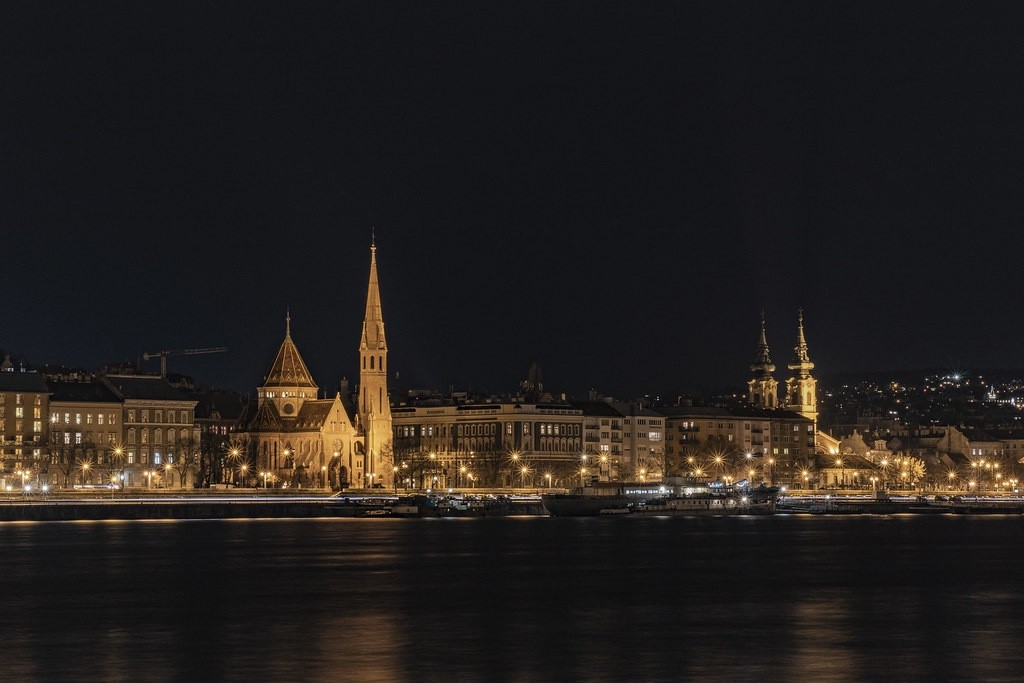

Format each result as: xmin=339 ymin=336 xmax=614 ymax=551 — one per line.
xmin=388 ymin=493 xmax=459 ymax=518
xmin=628 ymin=487 xmax=778 ymax=516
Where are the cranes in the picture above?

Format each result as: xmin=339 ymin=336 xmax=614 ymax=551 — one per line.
xmin=143 ymin=347 xmax=228 ymax=376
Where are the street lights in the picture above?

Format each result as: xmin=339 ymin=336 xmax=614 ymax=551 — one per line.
xmin=145 ymin=472 xmax=155 ymax=489
xmin=521 ymin=468 xmax=527 ymax=488
xmin=987 ymin=464 xmax=999 ymax=491
xmin=512 ymin=456 xmax=518 ymax=486
xmin=1010 ymin=480 xmax=1017 ymax=491
xmin=18 ymin=471 xmax=29 ymax=488
xmin=82 ymin=466 xmax=88 ymax=488
xmin=870 ymin=478 xmax=878 ymax=488
xmin=837 ymin=460 xmax=845 ymax=490
xmin=166 ymin=466 xmax=170 ymax=488
xmin=111 ymin=478 xmax=116 ymax=498
xmin=367 ymin=473 xmax=375 ymax=490
xmin=903 ymin=461 xmax=912 ymax=491
xmin=546 ymin=474 xmax=551 ymax=487
xmin=971 ymin=460 xmax=984 ymax=490
xmin=261 ymin=472 xmax=270 ymax=488
